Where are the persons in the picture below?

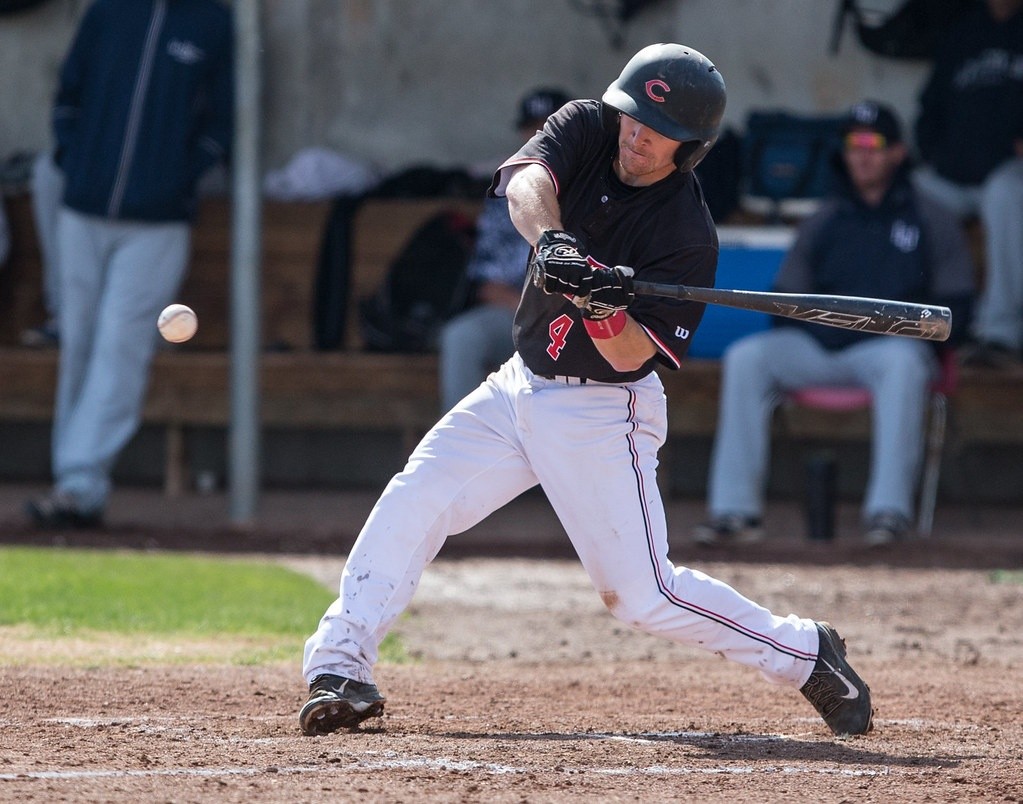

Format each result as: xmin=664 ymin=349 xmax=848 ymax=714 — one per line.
xmin=297 ymin=43 xmax=876 ymax=737
xmin=442 ymin=84 xmax=574 ymax=416
xmin=672 ymin=0 xmax=1023 ymax=544
xmin=25 ymin=0 xmax=237 ymax=528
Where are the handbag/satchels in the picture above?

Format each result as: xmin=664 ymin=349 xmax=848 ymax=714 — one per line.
xmin=741 ymin=104 xmax=852 ymax=200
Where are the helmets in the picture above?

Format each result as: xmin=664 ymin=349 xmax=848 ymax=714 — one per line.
xmin=599 ymin=42 xmax=726 ymax=174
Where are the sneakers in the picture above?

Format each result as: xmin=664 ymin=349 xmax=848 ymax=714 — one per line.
xmin=297 ymin=674 xmax=387 ymax=736
xmin=798 ymin=621 xmax=875 ymax=737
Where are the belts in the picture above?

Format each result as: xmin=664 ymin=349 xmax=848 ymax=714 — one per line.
xmin=523 ymin=363 xmax=586 ymax=386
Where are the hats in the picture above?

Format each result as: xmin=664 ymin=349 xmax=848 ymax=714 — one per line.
xmin=512 ymin=88 xmax=570 ymax=131
xmin=831 ymin=100 xmax=899 ymax=143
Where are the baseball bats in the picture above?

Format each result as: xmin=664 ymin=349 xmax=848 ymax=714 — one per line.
xmin=529 ymin=262 xmax=955 ymax=343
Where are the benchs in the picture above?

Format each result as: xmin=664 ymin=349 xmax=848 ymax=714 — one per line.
xmin=0 ymin=200 xmax=1023 ymax=538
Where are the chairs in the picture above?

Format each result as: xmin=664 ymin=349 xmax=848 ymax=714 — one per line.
xmin=791 ymin=359 xmax=956 ymax=545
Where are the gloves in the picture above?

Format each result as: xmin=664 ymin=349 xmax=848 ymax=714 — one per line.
xmin=531 ymin=230 xmax=593 ymax=309
xmin=579 ymin=265 xmax=636 ymax=323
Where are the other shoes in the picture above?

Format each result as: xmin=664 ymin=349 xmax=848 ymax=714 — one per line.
xmin=690 ymin=515 xmax=765 ymax=548
xmin=17 ymin=503 xmax=108 ymax=540
xmin=836 ymin=511 xmax=911 ymax=560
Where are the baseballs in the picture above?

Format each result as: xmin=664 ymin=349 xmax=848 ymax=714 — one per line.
xmin=157 ymin=302 xmax=199 ymax=344
